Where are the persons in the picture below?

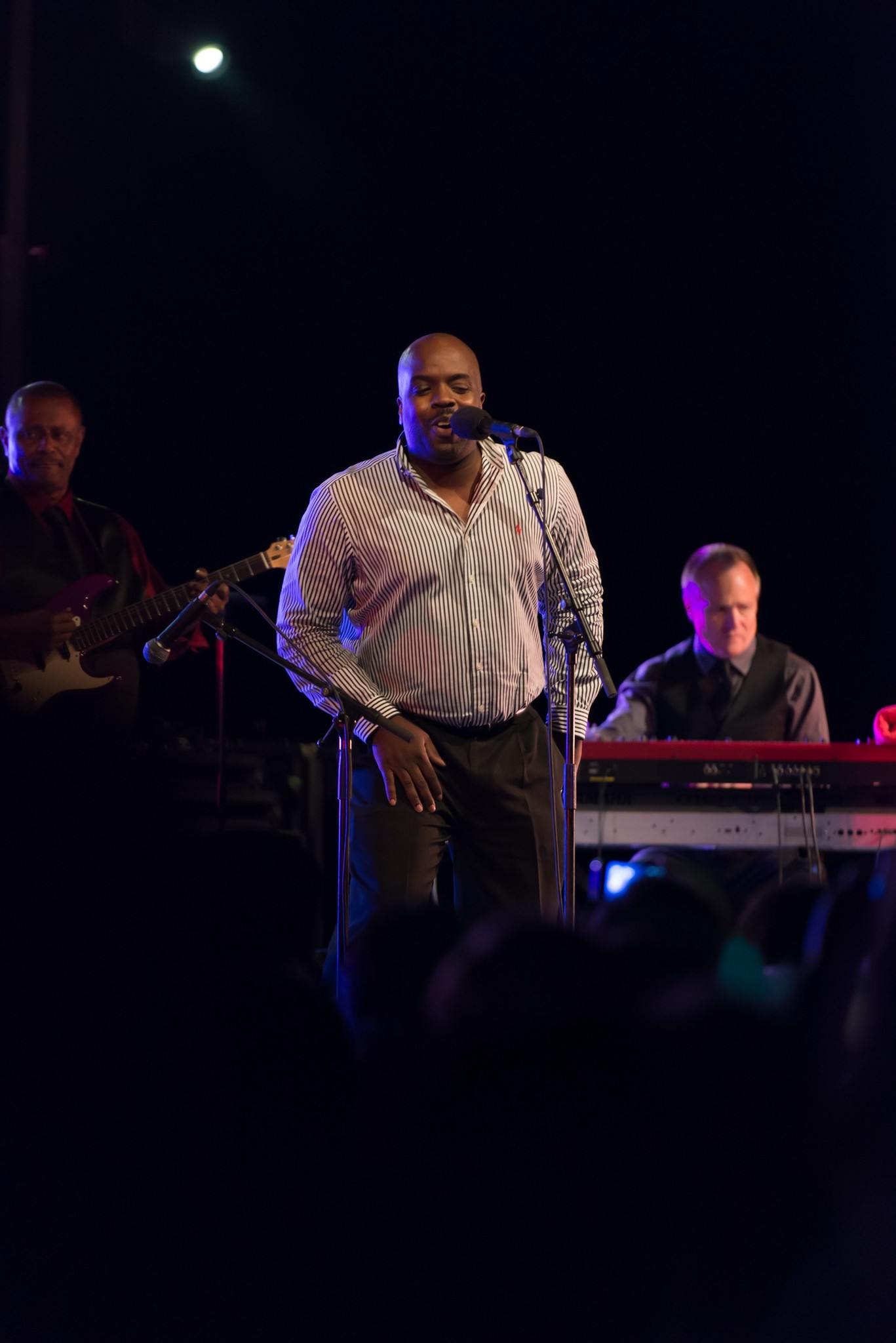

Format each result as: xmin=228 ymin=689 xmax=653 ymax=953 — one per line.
xmin=276 ymin=333 xmax=603 ymax=1014
xmin=0 ymin=380 xmax=230 ymax=711
xmin=324 ymin=870 xmax=896 ymax=1201
xmin=595 ymin=542 xmax=827 ymax=886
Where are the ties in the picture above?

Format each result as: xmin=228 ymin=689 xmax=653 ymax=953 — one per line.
xmin=43 ymin=507 xmax=86 ymax=574
xmin=711 ymin=658 xmax=732 ymax=728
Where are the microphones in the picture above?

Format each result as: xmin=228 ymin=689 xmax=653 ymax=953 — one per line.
xmin=143 ymin=581 xmax=222 ymax=665
xmin=450 ymin=406 xmax=539 ymax=439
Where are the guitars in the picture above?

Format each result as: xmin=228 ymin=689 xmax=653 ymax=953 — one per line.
xmin=0 ymin=533 xmax=295 ymax=718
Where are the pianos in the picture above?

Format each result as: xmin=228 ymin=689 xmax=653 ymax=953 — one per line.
xmin=575 ymin=734 xmax=896 ymax=849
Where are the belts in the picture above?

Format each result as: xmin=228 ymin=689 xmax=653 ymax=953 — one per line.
xmin=414 ymin=713 xmax=516 ymax=738
